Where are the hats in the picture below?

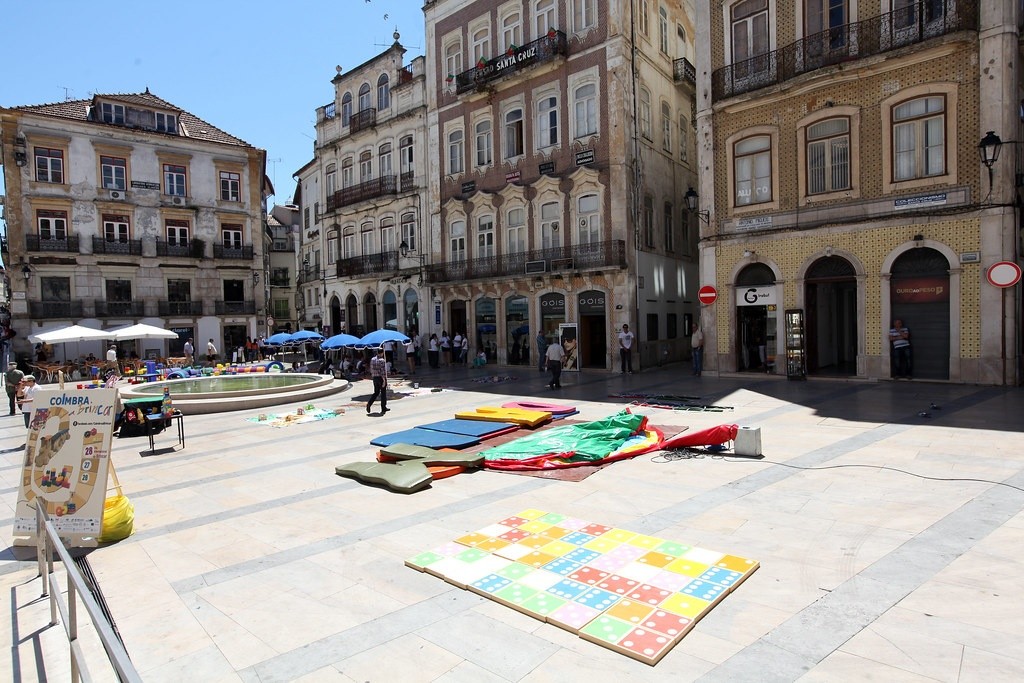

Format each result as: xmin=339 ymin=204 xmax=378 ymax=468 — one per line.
xmin=23 ymin=375 xmax=35 ymax=381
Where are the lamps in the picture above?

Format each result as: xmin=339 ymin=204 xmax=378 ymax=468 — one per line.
xmin=399 ymin=242 xmax=424 ymax=264
xmin=977 ymin=130 xmax=1002 ymax=191
xmin=913 ymin=235 xmax=925 ymax=242
xmin=303 ymin=259 xmax=310 ymax=272
xmin=20 ymin=266 xmax=32 ymax=289
xmin=253 ymin=271 xmax=260 ymax=287
xmin=682 ymin=188 xmax=711 ymax=226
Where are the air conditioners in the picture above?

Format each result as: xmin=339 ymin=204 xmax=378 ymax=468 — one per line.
xmin=171 ymin=195 xmax=186 ymax=206
xmin=109 ymin=190 xmax=126 ymax=201
xmin=262 ymin=213 xmax=267 ymax=221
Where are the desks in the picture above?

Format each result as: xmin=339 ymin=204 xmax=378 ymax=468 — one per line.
xmin=135 ymin=374 xmax=162 ymax=384
xmin=147 ymin=413 xmax=185 ymax=454
xmin=124 ymin=397 xmax=173 ymax=436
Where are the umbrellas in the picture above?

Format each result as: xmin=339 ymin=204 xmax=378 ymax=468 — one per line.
xmin=27 ymin=325 xmax=179 ymax=363
xmin=263 ymin=329 xmax=411 ymax=379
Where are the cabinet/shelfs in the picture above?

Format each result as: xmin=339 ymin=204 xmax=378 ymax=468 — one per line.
xmin=785 ymin=309 xmax=806 ymax=381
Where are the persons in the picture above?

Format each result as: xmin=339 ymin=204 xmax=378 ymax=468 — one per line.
xmin=4 ymin=362 xmax=42 ymax=446
xmin=79 ymin=344 xmax=139 ymax=380
xmin=691 ymin=322 xmax=704 ymax=376
xmin=537 ymin=330 xmax=547 ymax=373
xmin=888 ymin=318 xmax=913 ymax=380
xmin=183 ymin=336 xmax=399 ymax=412
xmin=618 ymin=324 xmax=635 ymax=375
xmin=545 ymin=338 xmax=566 ymax=389
xmin=401 ymin=331 xmax=488 ymax=368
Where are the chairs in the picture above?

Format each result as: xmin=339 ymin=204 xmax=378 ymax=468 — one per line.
xmin=25 ymin=361 xmax=72 ymax=383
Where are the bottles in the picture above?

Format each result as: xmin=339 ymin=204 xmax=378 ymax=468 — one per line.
xmin=162 ymin=386 xmax=172 ymax=417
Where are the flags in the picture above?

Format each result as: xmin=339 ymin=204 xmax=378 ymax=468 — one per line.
xmin=547 ymin=26 xmax=556 ymax=37
xmin=476 ymin=57 xmax=487 ymax=69
xmin=445 ymin=74 xmax=454 ymax=82
xmin=507 ymin=44 xmax=517 ymax=57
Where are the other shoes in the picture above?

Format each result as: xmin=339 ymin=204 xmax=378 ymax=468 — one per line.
xmin=696 ymin=374 xmax=700 ymax=376
xmin=628 ymin=371 xmax=632 ymax=374
xmin=382 ymin=408 xmax=390 ymax=411
xmin=549 ymin=383 xmax=553 ymax=389
xmin=366 ymin=404 xmax=370 ymax=413
xmin=620 ymin=371 xmax=624 ymax=375
